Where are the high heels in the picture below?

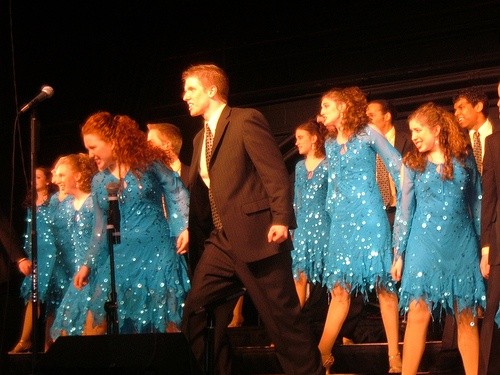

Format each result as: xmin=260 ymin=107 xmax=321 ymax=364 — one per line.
xmin=322 ymin=354 xmax=336 ymax=375
xmin=388 ymin=352 xmax=403 ymax=374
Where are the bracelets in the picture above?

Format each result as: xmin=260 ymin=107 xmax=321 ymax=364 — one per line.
xmin=18 ymin=258 xmax=27 ymax=264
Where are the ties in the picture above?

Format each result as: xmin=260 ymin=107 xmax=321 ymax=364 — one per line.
xmin=377 ymin=154 xmax=392 ymax=210
xmin=205 ymin=123 xmax=223 ymax=231
xmin=473 ymin=132 xmax=482 ymax=175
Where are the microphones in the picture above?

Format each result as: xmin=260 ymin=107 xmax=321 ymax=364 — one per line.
xmin=18 ymin=86 xmax=54 ymax=115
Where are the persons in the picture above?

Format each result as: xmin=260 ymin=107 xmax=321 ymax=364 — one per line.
xmin=182 ymin=64 xmax=329 ymax=375
xmin=0 ymin=81 xmax=500 ymax=375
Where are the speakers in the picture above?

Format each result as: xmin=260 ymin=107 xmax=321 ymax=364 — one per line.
xmin=34 ymin=333 xmax=200 ymax=375
